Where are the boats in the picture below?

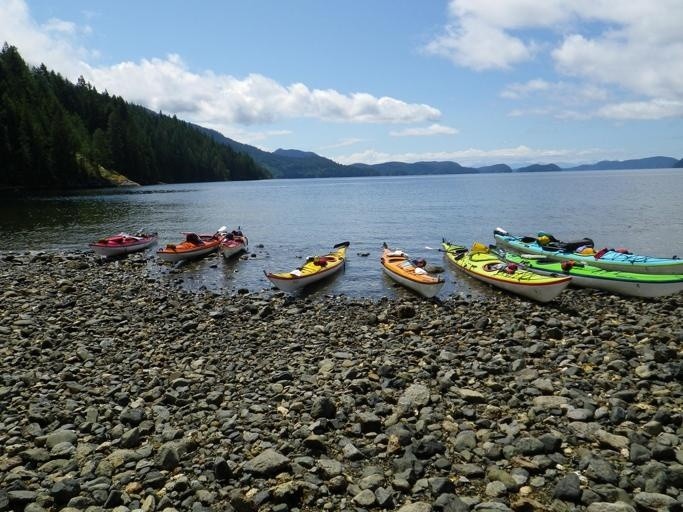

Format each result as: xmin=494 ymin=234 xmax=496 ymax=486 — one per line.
xmin=88 ymin=231 xmax=158 ymax=257
xmin=262 ymin=240 xmax=351 ymax=292
xmin=379 ymin=241 xmax=446 ymax=299
xmin=439 ymin=226 xmax=683 ymax=304
xmin=155 ymin=227 xmax=248 ymax=263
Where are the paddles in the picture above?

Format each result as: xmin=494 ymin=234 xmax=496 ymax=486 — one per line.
xmin=396 ymin=250 xmax=428 ymax=276
xmin=520 ymin=253 xmax=586 ymax=268
xmin=208 ymin=226 xmax=227 ymax=243
xmin=290 ymin=256 xmax=315 ymax=278
xmin=471 ymin=243 xmax=528 ymax=270
